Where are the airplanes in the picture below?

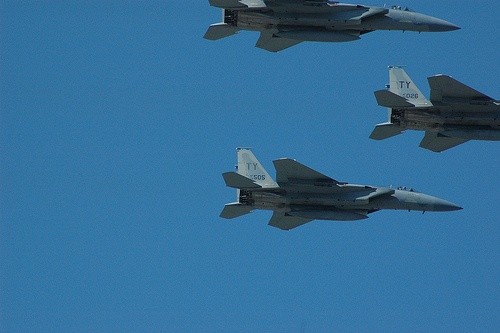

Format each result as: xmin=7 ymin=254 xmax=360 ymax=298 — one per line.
xmin=202 ymin=0 xmax=464 ymax=53
xmin=370 ymin=65 xmax=500 ymax=154
xmin=218 ymin=146 xmax=464 ymax=230
